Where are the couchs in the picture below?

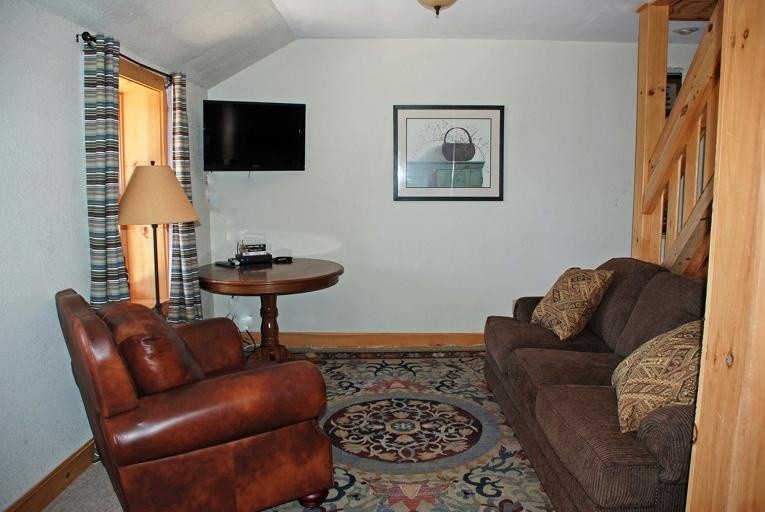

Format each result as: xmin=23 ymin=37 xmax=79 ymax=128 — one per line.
xmin=483 ymin=252 xmax=706 ymax=509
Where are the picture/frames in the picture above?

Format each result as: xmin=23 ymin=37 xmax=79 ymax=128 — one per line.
xmin=392 ymin=102 xmax=506 ymax=201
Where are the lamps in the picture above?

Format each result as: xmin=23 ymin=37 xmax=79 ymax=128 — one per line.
xmin=417 ymin=0 xmax=458 ymax=17
xmin=116 ymin=161 xmax=201 ymax=321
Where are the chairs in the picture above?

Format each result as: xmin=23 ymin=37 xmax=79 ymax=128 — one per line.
xmin=52 ymin=284 xmax=335 ymax=510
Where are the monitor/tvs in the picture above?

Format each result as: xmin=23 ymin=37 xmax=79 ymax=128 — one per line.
xmin=202 ymin=100 xmax=306 ymax=172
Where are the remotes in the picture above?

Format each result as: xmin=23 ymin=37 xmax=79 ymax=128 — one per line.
xmin=228 ymin=258 xmax=240 ymax=265
xmin=216 ymin=262 xmax=236 ymax=267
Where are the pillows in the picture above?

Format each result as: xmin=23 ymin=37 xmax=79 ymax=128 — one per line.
xmin=532 ymin=265 xmax=611 ymax=346
xmin=95 ymin=303 xmax=207 ymax=396
xmin=610 ymin=320 xmax=703 ymax=439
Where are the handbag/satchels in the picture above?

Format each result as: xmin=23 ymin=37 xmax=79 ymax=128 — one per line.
xmin=442 ymin=127 xmax=476 ymax=161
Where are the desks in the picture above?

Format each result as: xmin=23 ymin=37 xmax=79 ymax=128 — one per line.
xmin=198 ymin=256 xmax=345 ymax=367
